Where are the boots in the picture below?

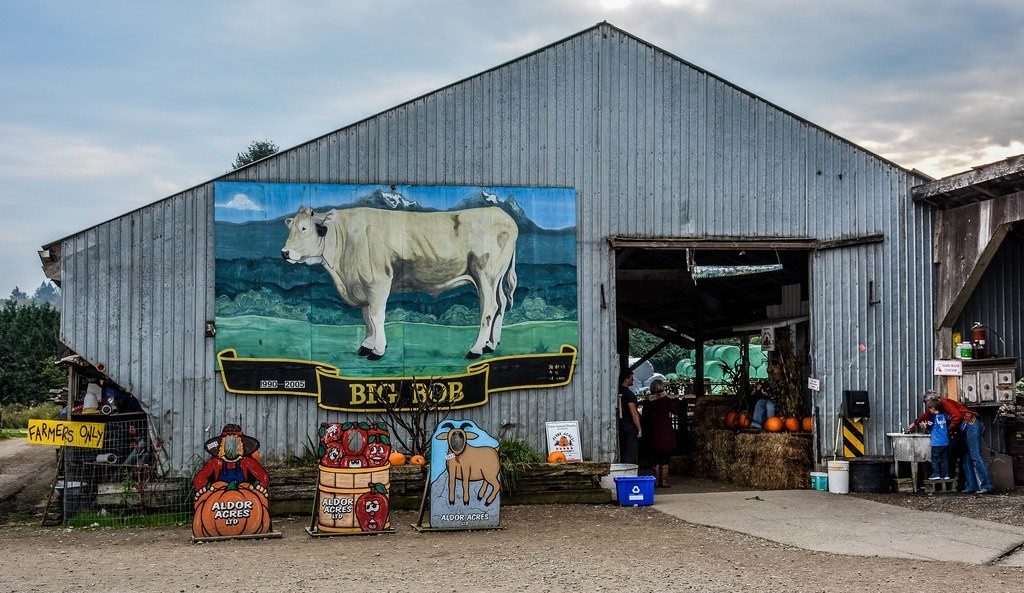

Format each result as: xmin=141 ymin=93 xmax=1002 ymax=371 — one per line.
xmin=662 ymin=465 xmax=671 ymax=488
xmin=654 ymin=465 xmax=661 ymax=487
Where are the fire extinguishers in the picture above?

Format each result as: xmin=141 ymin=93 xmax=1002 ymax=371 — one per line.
xmin=971 ymin=321 xmax=1005 ymax=359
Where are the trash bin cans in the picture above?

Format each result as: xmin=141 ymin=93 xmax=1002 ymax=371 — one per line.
xmin=613 ymin=476 xmax=656 ymax=506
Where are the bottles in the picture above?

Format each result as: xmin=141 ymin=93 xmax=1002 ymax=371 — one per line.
xmin=954 ymin=343 xmax=962 ymax=358
xmin=961 ymin=342 xmax=973 ymax=359
xmin=83 ymin=393 xmax=98 ymax=409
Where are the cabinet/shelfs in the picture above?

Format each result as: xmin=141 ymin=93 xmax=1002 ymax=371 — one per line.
xmin=945 ymin=365 xmax=1016 ymax=408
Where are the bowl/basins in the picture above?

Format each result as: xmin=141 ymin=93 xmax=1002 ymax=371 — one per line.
xmin=51 ymin=482 xmax=87 ymax=496
xmin=973 ymin=349 xmax=985 ymax=359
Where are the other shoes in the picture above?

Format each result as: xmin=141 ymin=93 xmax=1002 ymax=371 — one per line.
xmin=962 ymin=489 xmax=972 ymax=493
xmin=929 ymin=474 xmax=940 ymax=480
xmin=976 ymin=488 xmax=990 ymax=494
xmin=941 ymin=473 xmax=950 ymax=480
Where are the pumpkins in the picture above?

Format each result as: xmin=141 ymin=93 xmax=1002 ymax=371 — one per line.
xmin=725 ymin=411 xmax=750 ymax=427
xmin=547 ymin=451 xmax=566 ymax=463
xmin=251 ymin=451 xmax=259 ymax=462
xmin=410 ymin=455 xmax=425 ymax=465
xmin=764 ymin=416 xmax=812 ymax=431
xmin=388 ymin=449 xmax=406 ymax=465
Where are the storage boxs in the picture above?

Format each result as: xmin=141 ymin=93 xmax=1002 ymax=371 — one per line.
xmin=613 ymin=475 xmax=656 ymax=506
xmin=810 ymin=471 xmax=829 ymax=491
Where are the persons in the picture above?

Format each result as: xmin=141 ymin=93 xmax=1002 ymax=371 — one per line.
xmin=920 ymin=399 xmax=954 ymax=480
xmin=619 ymin=370 xmax=642 ymax=465
xmin=745 ymin=364 xmax=783 ymax=432
xmin=642 ymin=379 xmax=680 ymax=489
xmin=909 ymin=390 xmax=993 ymax=494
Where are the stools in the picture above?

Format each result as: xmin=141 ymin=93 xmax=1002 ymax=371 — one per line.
xmin=924 ymin=478 xmax=957 ymax=493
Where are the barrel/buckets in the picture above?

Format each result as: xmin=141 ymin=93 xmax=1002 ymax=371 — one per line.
xmin=599 ymin=463 xmax=639 ymax=500
xmin=810 ymin=471 xmax=828 ymax=491
xmin=850 ymin=460 xmax=892 ymax=494
xmin=827 ymin=460 xmax=850 ymax=494
